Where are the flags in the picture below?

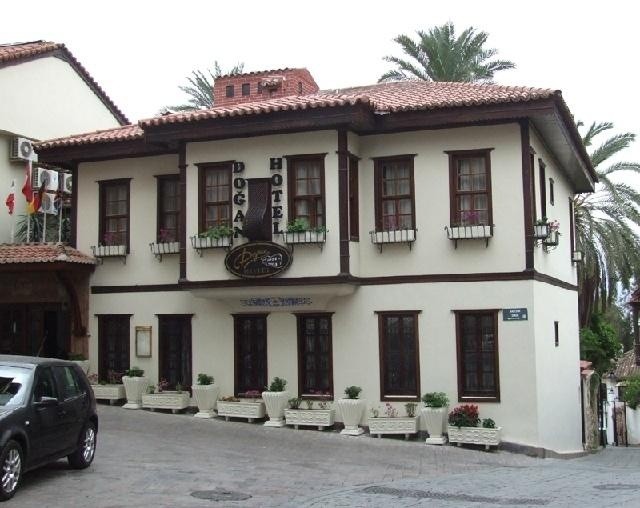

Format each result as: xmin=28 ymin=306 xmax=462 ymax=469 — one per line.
xmin=25 ymin=179 xmax=49 ymax=213
xmin=21 ymin=164 xmax=33 ymax=203
xmin=5 ymin=185 xmax=15 ymax=214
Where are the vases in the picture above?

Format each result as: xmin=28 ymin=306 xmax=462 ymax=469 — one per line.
xmin=94 ymin=245 xmax=127 ymax=257
xmin=448 ymin=226 xmax=491 ymax=240
xmin=153 ymin=242 xmax=180 ymax=254
xmin=216 ymin=401 xmax=266 ymax=422
xmin=448 ymin=423 xmax=503 ymax=450
xmin=542 ymin=232 xmax=558 ymax=245
xmin=372 ymin=230 xmax=415 ymax=243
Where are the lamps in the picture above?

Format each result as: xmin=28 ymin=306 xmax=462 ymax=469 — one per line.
xmin=135 ymin=326 xmax=152 ymax=358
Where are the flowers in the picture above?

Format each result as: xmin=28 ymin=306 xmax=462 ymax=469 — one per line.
xmin=244 ymin=390 xmax=261 ymax=403
xmin=158 ymin=229 xmax=174 ymax=243
xmin=547 ymin=219 xmax=560 ymax=233
xmin=447 ymin=404 xmax=482 ymax=427
xmin=385 ymin=216 xmax=400 ymax=231
xmin=104 ymin=232 xmax=120 ymax=246
xmin=462 ymin=208 xmax=479 ymax=225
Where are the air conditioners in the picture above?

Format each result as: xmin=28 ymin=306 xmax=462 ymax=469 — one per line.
xmin=573 ymin=250 xmax=585 ymax=262
xmin=32 ymin=167 xmax=72 ymax=216
xmin=9 ymin=137 xmax=39 ymax=165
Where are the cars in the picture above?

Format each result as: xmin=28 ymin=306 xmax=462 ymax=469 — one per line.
xmin=1 ymin=354 xmax=100 ymax=502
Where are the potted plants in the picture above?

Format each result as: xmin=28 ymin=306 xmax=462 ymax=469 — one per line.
xmin=67 ymin=354 xmax=219 ymax=420
xmin=261 ymin=376 xmax=450 ymax=445
xmin=534 ymin=217 xmax=549 ymax=235
xmin=284 ymin=217 xmax=328 ymax=243
xmin=192 ymin=225 xmax=234 ymax=248
